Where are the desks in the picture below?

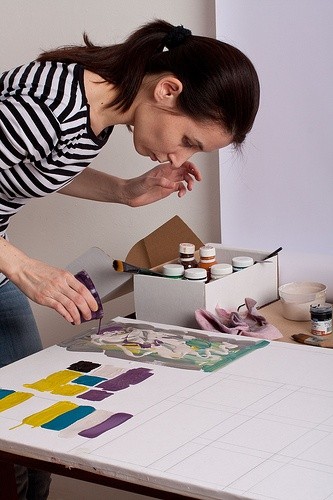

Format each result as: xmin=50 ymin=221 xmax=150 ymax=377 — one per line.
xmin=0 ymin=299 xmax=333 ymax=500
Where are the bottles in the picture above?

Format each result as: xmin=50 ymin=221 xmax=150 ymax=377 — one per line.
xmin=197 ymin=247 xmax=218 ymax=283
xmin=208 ymin=263 xmax=233 ymax=283
xmin=177 ymin=242 xmax=198 ymax=269
xmin=232 ymin=256 xmax=253 ymax=274
xmin=162 ymin=263 xmax=184 ymax=280
xmin=183 ymin=269 xmax=207 ymax=284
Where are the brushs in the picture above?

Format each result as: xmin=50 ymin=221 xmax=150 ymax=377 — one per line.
xmin=292 ymin=333 xmax=333 ymax=348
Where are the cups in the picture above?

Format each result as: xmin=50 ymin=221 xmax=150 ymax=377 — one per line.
xmin=310 ymin=302 xmax=333 ymax=336
xmin=64 ymin=270 xmax=105 ymax=325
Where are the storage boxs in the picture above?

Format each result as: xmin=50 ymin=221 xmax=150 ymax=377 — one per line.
xmin=64 ymin=215 xmax=280 ymax=328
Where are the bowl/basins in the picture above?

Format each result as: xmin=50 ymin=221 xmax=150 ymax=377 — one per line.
xmin=278 ymin=281 xmax=328 ymax=321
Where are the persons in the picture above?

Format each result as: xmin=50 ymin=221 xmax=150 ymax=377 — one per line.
xmin=0 ymin=19 xmax=260 ymax=500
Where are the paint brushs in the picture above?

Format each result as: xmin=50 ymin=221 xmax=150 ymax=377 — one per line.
xmin=112 ymin=259 xmax=160 ymax=276
xmin=254 ymin=247 xmax=282 ymax=264
